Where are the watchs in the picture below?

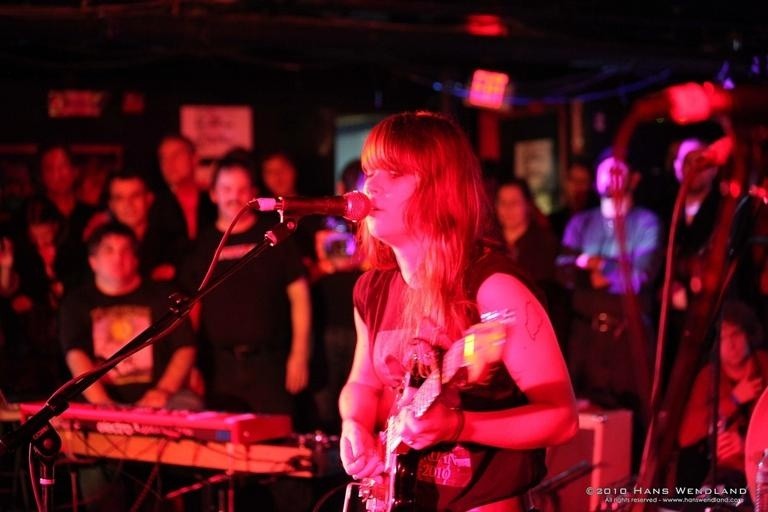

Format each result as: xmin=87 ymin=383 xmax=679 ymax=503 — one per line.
xmin=448 ymin=405 xmax=465 ymax=448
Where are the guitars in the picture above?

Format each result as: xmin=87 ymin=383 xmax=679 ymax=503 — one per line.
xmin=361 ymin=311 xmax=511 ymax=512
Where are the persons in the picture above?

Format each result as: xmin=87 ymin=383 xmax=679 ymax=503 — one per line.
xmin=337 ymin=111 xmax=579 ymax=512
xmin=678 ymin=303 xmax=763 ymax=512
xmin=334 ymin=160 xmax=367 ymax=194
xmin=261 ymin=153 xmax=308 ymax=251
xmin=663 ymin=137 xmax=726 ymax=276
xmin=191 ymin=159 xmax=312 ymax=512
xmin=491 ymin=178 xmax=555 ymax=325
xmin=58 ymin=223 xmax=208 ymax=512
xmin=558 ymin=153 xmax=661 ymax=488
xmin=551 ymin=164 xmax=598 ymax=239
xmin=0 ymin=146 xmax=111 ymax=367
xmin=106 ymin=171 xmax=184 ymax=292
xmin=154 ymin=135 xmax=217 ymax=259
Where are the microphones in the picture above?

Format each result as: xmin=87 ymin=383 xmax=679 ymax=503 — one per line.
xmin=255 ymin=190 xmax=372 ymax=223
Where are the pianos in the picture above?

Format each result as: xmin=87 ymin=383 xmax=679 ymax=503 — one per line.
xmin=16 ymin=401 xmax=294 ymax=446
